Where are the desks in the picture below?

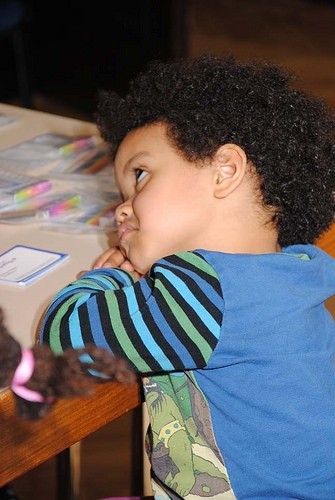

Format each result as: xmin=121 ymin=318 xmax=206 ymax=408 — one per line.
xmin=0 ymin=104 xmax=335 ymax=487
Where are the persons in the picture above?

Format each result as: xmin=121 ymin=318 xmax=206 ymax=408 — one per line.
xmin=36 ymin=51 xmax=335 ymax=500
